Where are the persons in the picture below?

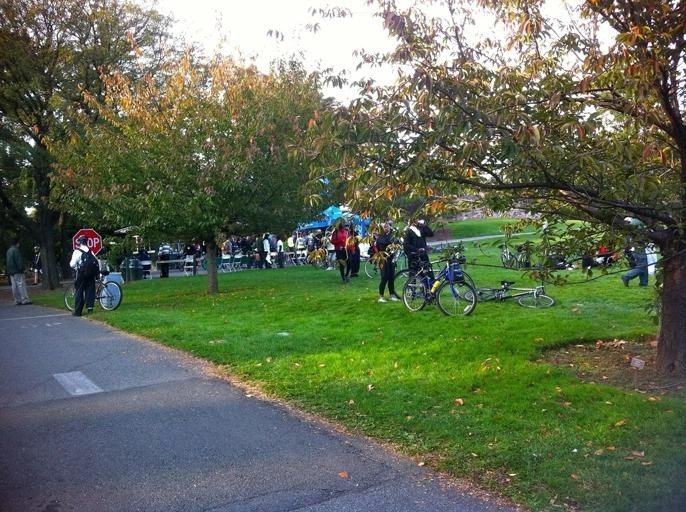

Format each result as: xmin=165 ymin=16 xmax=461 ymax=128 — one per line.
xmin=96 ymin=236 xmax=204 ymax=284
xmin=69 ymin=236 xmax=99 ymax=316
xmin=548 ymin=210 xmax=660 ymax=286
xmin=32 ymin=246 xmax=43 ymax=284
xmin=7 ymin=239 xmax=34 ymax=304
xmin=217 ymin=218 xmax=436 ymax=304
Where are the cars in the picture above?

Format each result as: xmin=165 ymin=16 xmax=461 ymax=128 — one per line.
xmin=158 ymin=246 xmax=173 ymax=256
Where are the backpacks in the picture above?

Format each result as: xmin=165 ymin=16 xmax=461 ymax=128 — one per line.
xmin=75 ymin=247 xmax=99 ymax=269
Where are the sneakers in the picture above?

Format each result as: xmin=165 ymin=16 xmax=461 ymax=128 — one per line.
xmin=621 ymin=275 xmax=629 ymax=288
xmin=378 ymin=296 xmax=388 ymax=303
xmin=390 ymin=297 xmax=400 ymax=302
xmin=16 ymin=301 xmax=34 ymax=305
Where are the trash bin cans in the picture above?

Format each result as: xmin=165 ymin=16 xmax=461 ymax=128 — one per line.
xmin=129 ymin=259 xmax=143 ymax=280
xmin=100 ymin=273 xmax=122 ymax=305
xmin=120 ymin=261 xmax=126 ymax=281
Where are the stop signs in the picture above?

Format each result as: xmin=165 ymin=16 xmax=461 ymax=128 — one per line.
xmin=72 ymin=229 xmax=102 ymax=258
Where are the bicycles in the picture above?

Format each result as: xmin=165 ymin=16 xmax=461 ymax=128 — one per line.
xmin=495 ymin=237 xmax=532 ymax=271
xmin=363 ymin=244 xmax=477 ymax=318
xmin=60 ymin=266 xmax=124 ymax=313
xmin=463 ymin=277 xmax=557 ymax=309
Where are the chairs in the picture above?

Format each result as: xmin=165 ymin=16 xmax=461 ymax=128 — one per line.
xmin=182 ymin=254 xmax=197 ymax=276
xmin=220 ymin=253 xmax=231 ymax=274
xmin=232 ymin=253 xmax=243 ymax=272
xmin=266 ymin=250 xmax=307 ymax=268
xmin=140 ymin=260 xmax=153 ymax=280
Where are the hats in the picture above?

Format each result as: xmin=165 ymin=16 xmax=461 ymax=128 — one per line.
xmin=78 ymin=235 xmax=88 ymax=245
